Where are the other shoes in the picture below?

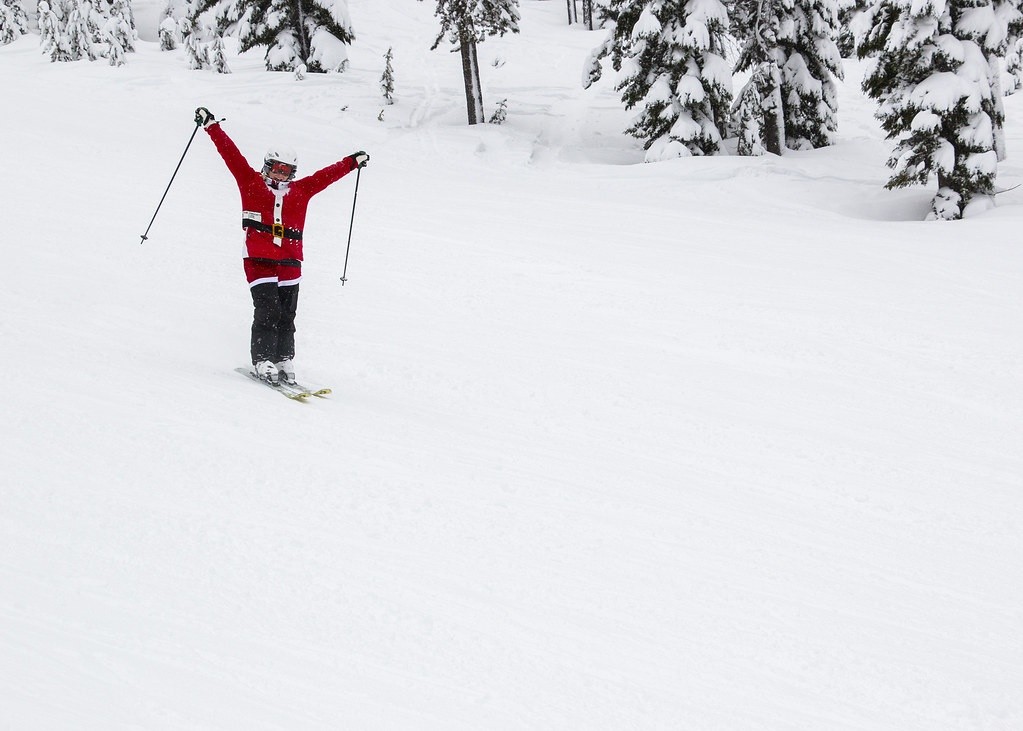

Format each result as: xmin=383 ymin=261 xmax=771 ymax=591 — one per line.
xmin=255 ymin=359 xmax=278 ymax=383
xmin=274 ymin=359 xmax=295 ymax=385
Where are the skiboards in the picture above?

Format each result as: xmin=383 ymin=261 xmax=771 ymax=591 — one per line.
xmin=233 ymin=362 xmax=333 ymax=400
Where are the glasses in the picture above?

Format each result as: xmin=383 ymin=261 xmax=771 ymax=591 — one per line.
xmin=270 ymin=161 xmax=293 ymax=176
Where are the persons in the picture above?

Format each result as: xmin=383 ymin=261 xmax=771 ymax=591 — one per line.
xmin=194 ymin=107 xmax=370 ymax=386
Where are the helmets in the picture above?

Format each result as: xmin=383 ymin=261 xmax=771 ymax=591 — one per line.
xmin=261 ymin=144 xmax=298 ymax=180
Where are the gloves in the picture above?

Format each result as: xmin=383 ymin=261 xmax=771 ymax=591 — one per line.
xmin=350 ymin=151 xmax=369 ymax=168
xmin=194 ymin=107 xmax=215 ymax=127
